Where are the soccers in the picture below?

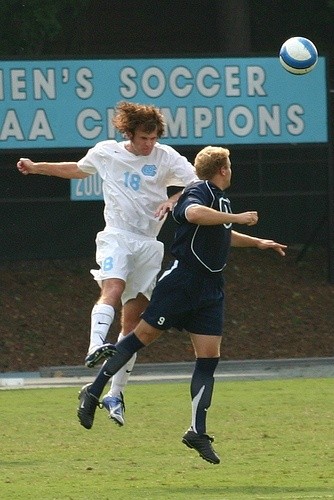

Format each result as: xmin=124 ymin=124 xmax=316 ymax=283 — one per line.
xmin=279 ymin=36 xmax=319 ymax=76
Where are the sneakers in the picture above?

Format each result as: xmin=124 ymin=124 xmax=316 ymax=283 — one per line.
xmin=102 ymin=391 xmax=126 ymax=426
xmin=85 ymin=343 xmax=118 ymax=369
xmin=77 ymin=382 xmax=100 ymax=429
xmin=181 ymin=425 xmax=220 ymax=465
